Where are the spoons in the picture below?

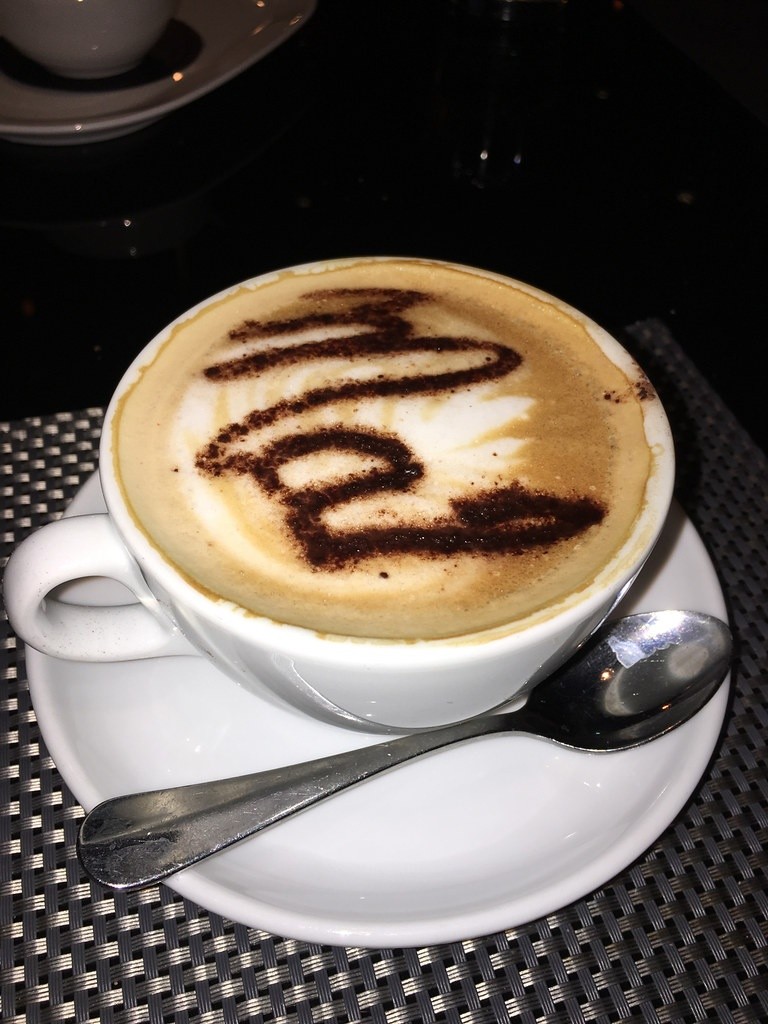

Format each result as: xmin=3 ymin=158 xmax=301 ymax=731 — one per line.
xmin=78 ymin=608 xmax=730 ymax=889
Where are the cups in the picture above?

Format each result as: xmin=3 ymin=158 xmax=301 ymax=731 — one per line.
xmin=0 ymin=252 xmax=676 ymax=736
xmin=0 ymin=0 xmax=175 ymax=78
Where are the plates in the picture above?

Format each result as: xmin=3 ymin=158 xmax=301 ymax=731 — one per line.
xmin=29 ymin=461 xmax=730 ymax=949
xmin=0 ymin=0 xmax=318 ymax=144
